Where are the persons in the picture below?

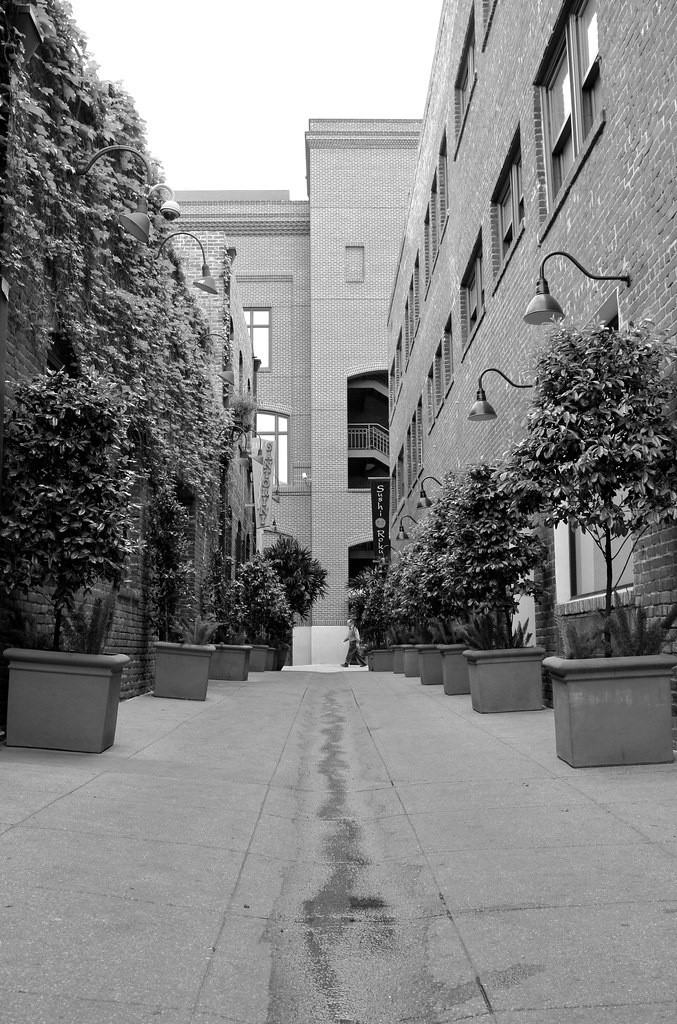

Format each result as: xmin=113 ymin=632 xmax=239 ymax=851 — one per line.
xmin=340 ymin=619 xmax=367 ymax=667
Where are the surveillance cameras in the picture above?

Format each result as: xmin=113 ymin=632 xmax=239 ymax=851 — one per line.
xmin=302 ymin=473 xmax=307 ymax=479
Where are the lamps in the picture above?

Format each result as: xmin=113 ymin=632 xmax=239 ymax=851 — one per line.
xmin=380 ymin=545 xmax=396 ymax=566
xmin=468 ymin=367 xmax=539 ymax=421
xmin=272 ymin=476 xmax=279 ymax=504
xmin=71 ymin=145 xmax=151 ymax=243
xmin=522 ymin=251 xmax=631 ymax=326
xmin=417 ymin=476 xmax=442 ymax=509
xmin=196 ymin=334 xmax=235 ymax=386
xmin=154 ymin=232 xmax=217 ymax=294
xmin=217 ymin=425 xmax=250 ymax=470
xmin=232 ymin=429 xmax=263 ymax=465
xmin=395 ymin=514 xmax=418 ymax=541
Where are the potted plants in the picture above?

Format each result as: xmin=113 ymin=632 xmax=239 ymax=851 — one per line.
xmin=248 ymin=633 xmax=280 ymax=671
xmin=4 ymin=591 xmax=131 ymax=753
xmin=542 ymin=588 xmax=677 ymax=769
xmin=366 ymin=611 xmax=546 ymax=714
xmin=206 ymin=626 xmax=253 ymax=680
xmin=153 ymin=612 xmax=223 ymax=701
